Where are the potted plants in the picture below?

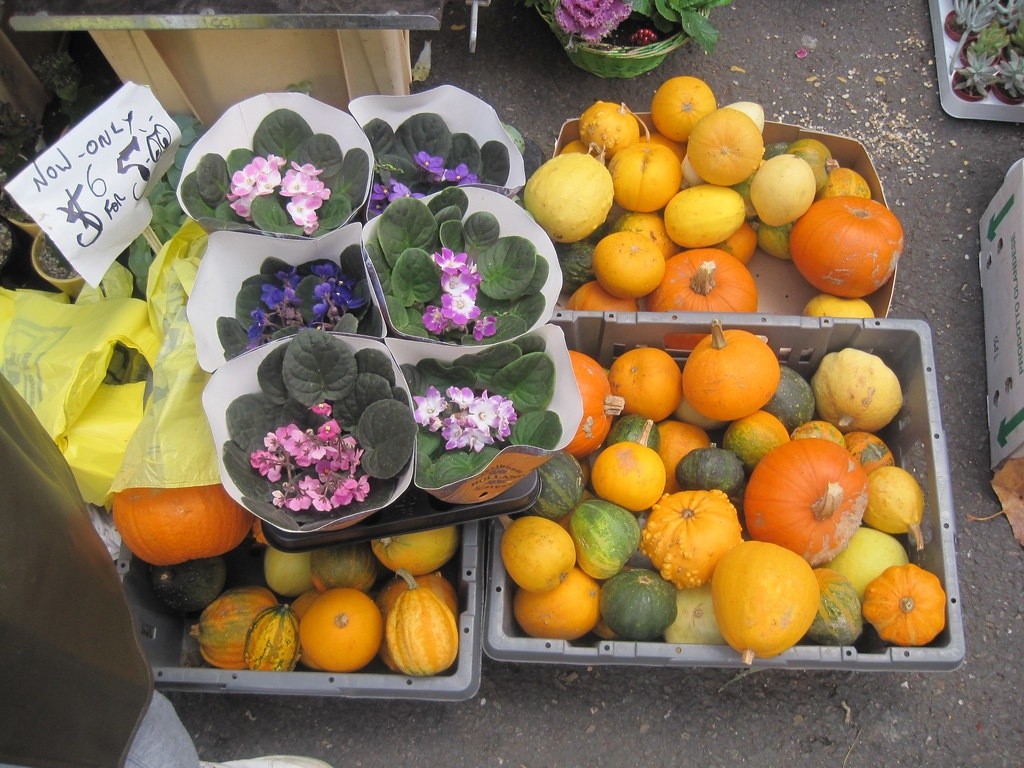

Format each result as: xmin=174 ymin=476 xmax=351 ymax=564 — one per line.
xmin=31 ymin=230 xmax=85 ymax=301
xmin=33 ymin=51 xmax=102 ymax=139
xmin=0 ymin=101 xmax=44 ymax=239
xmin=944 ymin=0 xmax=1024 ymax=105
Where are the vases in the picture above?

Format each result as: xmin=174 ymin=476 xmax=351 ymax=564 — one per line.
xmin=0 ymin=217 xmax=43 ymax=282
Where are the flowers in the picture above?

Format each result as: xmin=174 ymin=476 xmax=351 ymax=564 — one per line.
xmin=553 ymin=0 xmax=636 ymax=45
xmin=408 ymin=375 xmax=521 ymax=452
xmin=225 ymin=145 xmax=333 ymax=237
xmin=243 ymin=261 xmax=370 ymax=351
xmin=364 ymin=141 xmax=486 ymax=220
xmin=414 ymin=246 xmax=495 ymax=343
xmin=248 ymin=401 xmax=373 ymax=514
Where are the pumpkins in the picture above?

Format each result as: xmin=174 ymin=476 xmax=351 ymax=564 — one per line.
xmin=522 ymin=76 xmax=905 ymax=351
xmin=498 ymin=319 xmax=946 ymax=665
xmin=109 ymin=483 xmax=461 ymax=677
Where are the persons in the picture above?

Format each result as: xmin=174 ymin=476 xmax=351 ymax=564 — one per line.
xmin=0 ymin=374 xmax=331 ymax=768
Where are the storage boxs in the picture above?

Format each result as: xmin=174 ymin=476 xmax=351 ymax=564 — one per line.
xmin=978 ymin=157 xmax=1024 ymax=475
xmin=552 ymin=115 xmax=897 ymax=362
xmin=85 ymin=29 xmax=414 ymax=129
xmin=480 ymin=311 xmax=967 ymax=671
xmin=118 ymin=518 xmax=488 ymax=703
